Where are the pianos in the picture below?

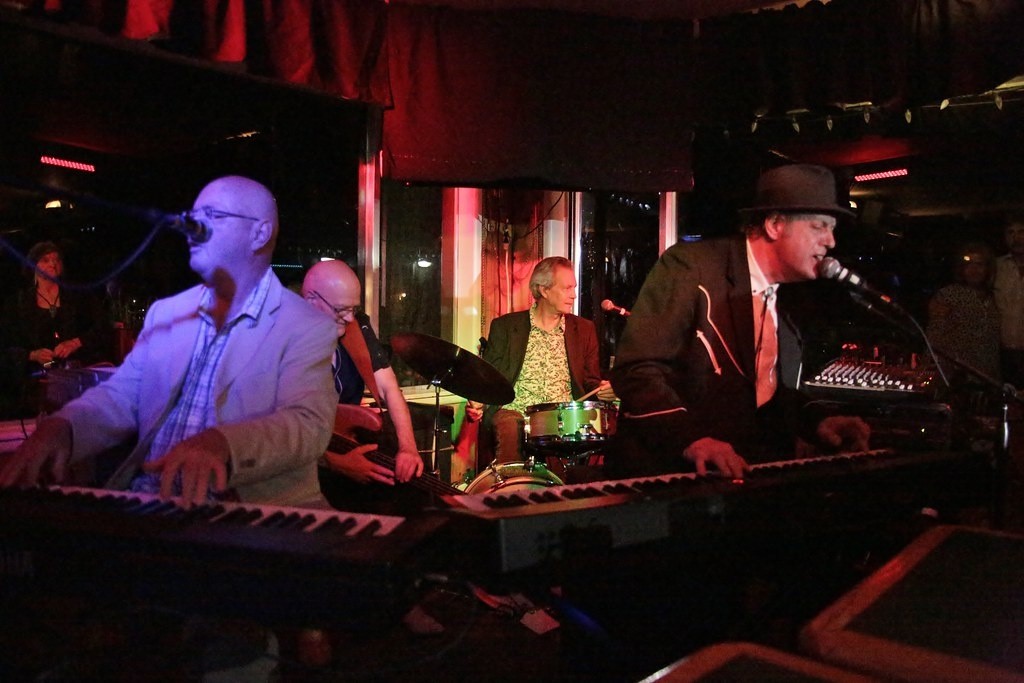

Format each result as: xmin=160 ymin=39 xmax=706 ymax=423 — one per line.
xmin=0 ymin=482 xmax=455 ymax=683
xmin=434 ymin=431 xmax=1013 ymax=682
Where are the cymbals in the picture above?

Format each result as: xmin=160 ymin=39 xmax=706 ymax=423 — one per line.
xmin=388 ymin=330 xmax=517 ymax=406
xmin=397 ymin=381 xmax=477 ymax=409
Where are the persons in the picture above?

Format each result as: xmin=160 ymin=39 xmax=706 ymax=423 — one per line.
xmin=925 ymin=211 xmax=1024 ymax=396
xmin=0 ymin=241 xmax=100 ymax=417
xmin=617 ymin=164 xmax=871 ymax=479
xmin=299 ymin=260 xmax=423 ymax=512
xmin=465 ymin=258 xmax=617 ymax=484
xmin=0 ymin=176 xmax=341 ymax=512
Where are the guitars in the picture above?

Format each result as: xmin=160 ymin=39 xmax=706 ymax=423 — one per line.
xmin=325 ymin=401 xmax=467 ymax=507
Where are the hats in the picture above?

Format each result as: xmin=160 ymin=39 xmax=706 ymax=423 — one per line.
xmin=737 ymin=162 xmax=860 ymax=223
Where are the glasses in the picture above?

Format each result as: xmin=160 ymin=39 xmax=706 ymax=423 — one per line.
xmin=308 ymin=288 xmax=362 ymax=317
xmin=189 ymin=208 xmax=261 ymax=223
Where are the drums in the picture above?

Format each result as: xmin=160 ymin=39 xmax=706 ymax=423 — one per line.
xmin=526 ymin=399 xmax=618 ymax=441
xmin=458 ymin=455 xmax=569 ymax=612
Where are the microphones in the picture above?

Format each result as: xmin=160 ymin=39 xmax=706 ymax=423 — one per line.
xmin=819 ymin=257 xmax=892 ymax=308
xmin=134 ymin=204 xmax=213 ymax=243
xmin=600 ymin=299 xmax=631 ymax=317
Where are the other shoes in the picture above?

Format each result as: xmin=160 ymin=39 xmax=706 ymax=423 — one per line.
xmin=402 ymin=605 xmax=445 ymax=637
xmin=297 ymin=626 xmax=333 ymax=672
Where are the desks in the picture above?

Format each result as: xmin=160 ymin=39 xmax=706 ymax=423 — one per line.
xmin=635 ymin=639 xmax=869 ymax=683
xmin=802 ymin=523 xmax=1024 ymax=683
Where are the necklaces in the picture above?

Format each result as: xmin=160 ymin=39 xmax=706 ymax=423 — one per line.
xmin=37 ymin=290 xmax=59 ymax=319
